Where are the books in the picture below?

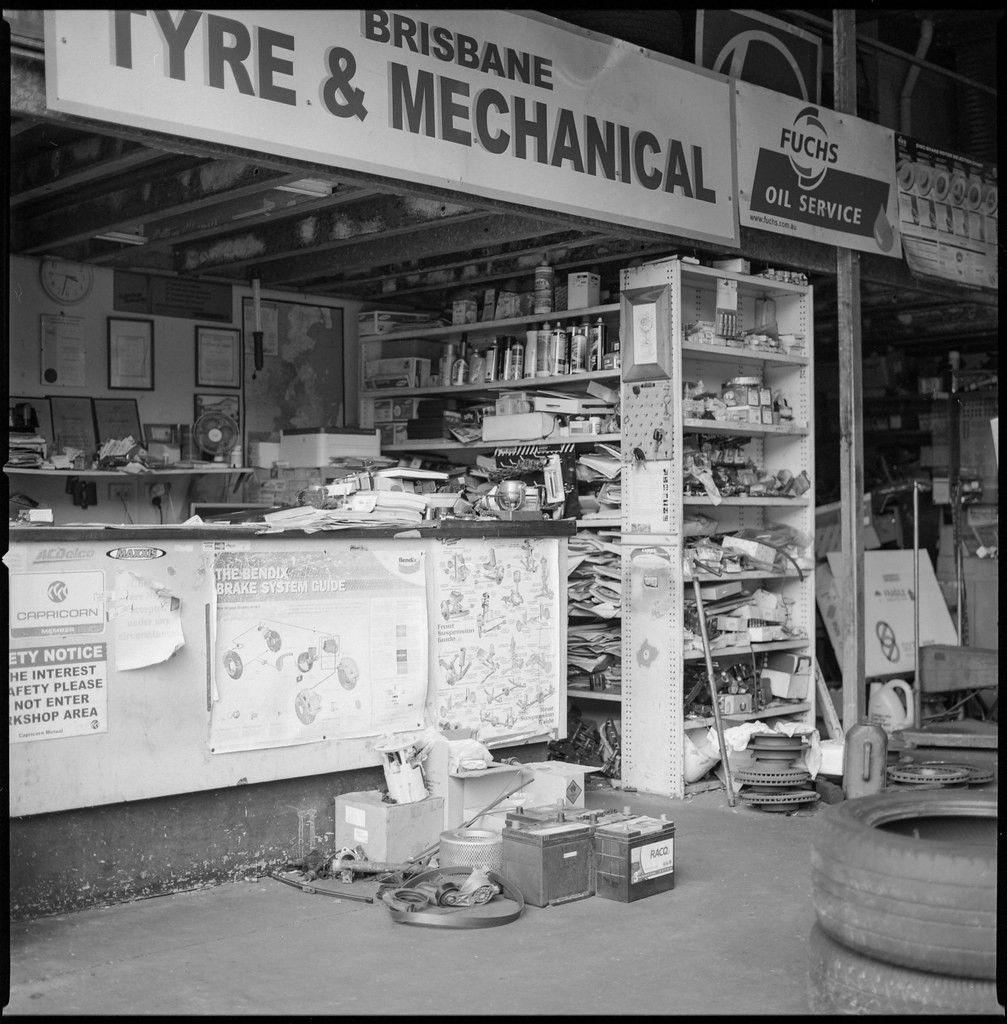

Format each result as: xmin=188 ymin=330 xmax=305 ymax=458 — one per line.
xmin=198 ymin=461 xmax=450 ymax=532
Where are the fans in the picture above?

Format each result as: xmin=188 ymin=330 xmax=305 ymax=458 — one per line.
xmin=193 ymin=411 xmax=238 ymax=454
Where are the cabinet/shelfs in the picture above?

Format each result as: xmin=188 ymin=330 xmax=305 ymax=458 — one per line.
xmin=815 ymin=301 xmax=999 ymax=549
xmin=354 ymin=257 xmax=623 ymax=800
xmin=621 ymin=260 xmax=815 ymax=800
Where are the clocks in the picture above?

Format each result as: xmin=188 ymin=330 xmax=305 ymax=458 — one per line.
xmin=40 ymin=257 xmax=94 ymax=306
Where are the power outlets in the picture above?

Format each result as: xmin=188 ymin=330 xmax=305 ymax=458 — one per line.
xmin=110 ymin=484 xmax=133 ymax=501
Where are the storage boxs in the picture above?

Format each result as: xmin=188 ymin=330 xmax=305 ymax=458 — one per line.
xmin=249 ymin=427 xmax=380 ymax=468
xmin=335 ymin=729 xmax=605 ymax=871
xmin=358 ymin=311 xmax=430 ymax=445
xmin=454 ymin=272 xmax=999 ymax=699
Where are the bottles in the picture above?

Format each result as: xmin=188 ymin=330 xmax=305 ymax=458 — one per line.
xmin=437 ymin=251 xmax=608 ymax=386
